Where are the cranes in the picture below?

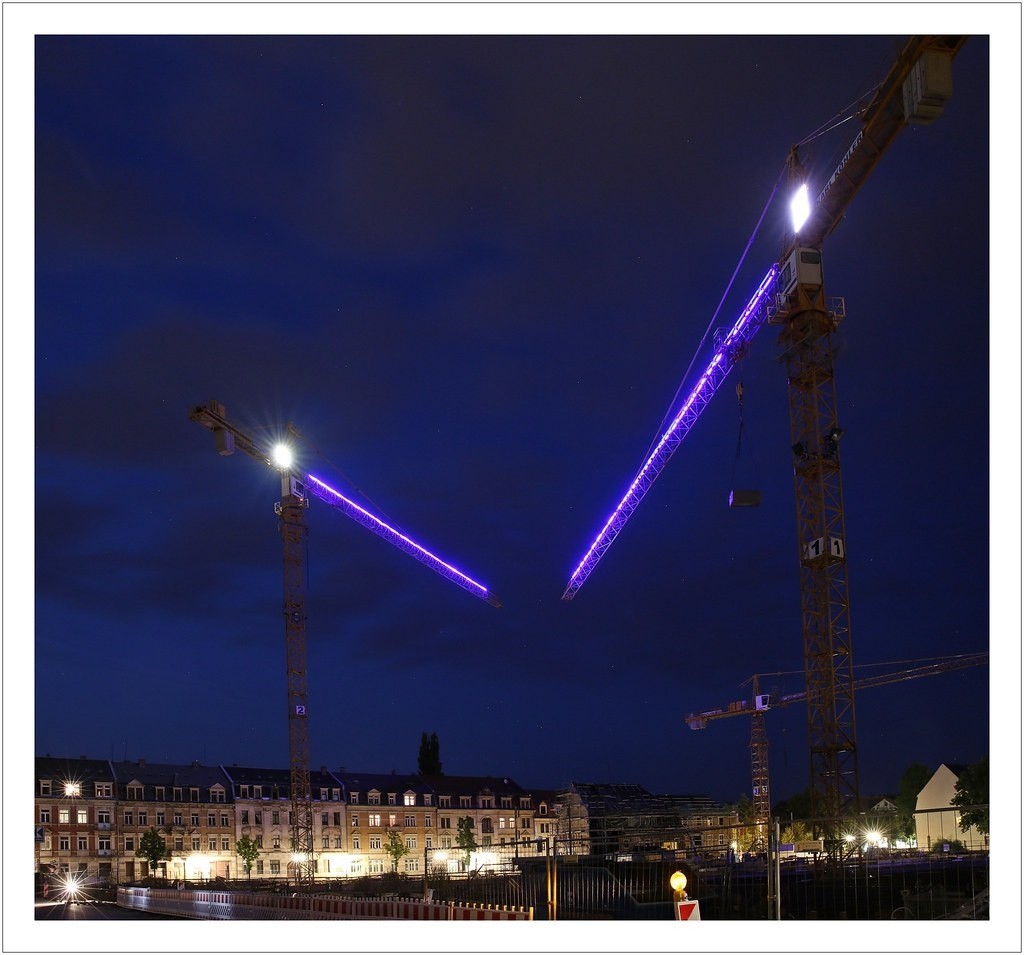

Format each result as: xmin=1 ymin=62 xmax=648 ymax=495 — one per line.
xmin=192 ymin=399 xmax=498 ymax=891
xmin=554 ymin=33 xmax=966 ymax=801
xmin=682 ymin=653 xmax=989 ymax=851
